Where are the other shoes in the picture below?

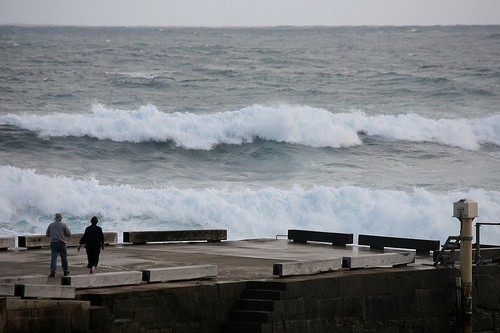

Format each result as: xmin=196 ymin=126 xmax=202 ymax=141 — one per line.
xmin=64 ymin=271 xmax=69 ymax=276
xmin=90 ymin=269 xmax=94 ymax=274
xmin=49 ymin=271 xmax=55 ymax=277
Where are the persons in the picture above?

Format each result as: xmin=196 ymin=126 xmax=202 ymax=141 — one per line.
xmin=77 ymin=216 xmax=105 ymax=274
xmin=45 ymin=213 xmax=72 ymax=277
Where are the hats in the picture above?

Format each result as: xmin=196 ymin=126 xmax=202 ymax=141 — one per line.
xmin=54 ymin=213 xmax=63 ymax=220
